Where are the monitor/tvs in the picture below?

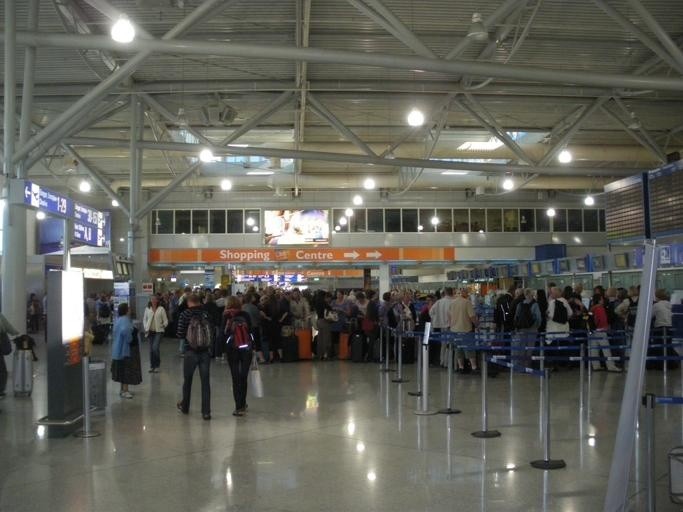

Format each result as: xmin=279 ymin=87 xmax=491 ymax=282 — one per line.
xmin=559 ymin=260 xmax=569 ymax=271
xmin=581 ymin=298 xmax=589 ymax=310
xmin=669 ymin=288 xmax=682 ymax=306
xmin=545 ymin=262 xmax=554 ymax=273
xmin=259 ymin=206 xmax=332 ymax=247
xmin=530 ymin=263 xmax=541 ymax=273
xmin=592 ymin=255 xmax=605 ymax=268
xmin=575 ymin=257 xmax=587 ymax=270
xmin=521 ymin=264 xmax=528 ymax=274
xmin=614 ymin=252 xmax=629 ymax=268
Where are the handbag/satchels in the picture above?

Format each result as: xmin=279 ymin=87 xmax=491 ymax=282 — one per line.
xmin=248 ymin=349 xmax=264 ymax=398
xmin=324 ymin=308 xmax=339 ymax=321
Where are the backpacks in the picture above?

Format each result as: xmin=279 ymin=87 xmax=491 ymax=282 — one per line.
xmin=494 ymin=294 xmax=637 ymax=329
xmin=224 ymin=315 xmax=254 ymax=352
xmin=98 ymin=302 xmax=110 ymax=318
xmin=185 ymin=314 xmax=216 ymax=352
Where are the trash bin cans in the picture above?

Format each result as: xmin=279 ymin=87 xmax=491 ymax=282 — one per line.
xmin=89 ymin=360 xmax=107 ymax=410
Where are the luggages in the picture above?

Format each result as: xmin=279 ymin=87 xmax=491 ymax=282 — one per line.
xmin=91 ymin=325 xmax=107 ymax=344
xmin=281 ymin=320 xmax=415 ymax=365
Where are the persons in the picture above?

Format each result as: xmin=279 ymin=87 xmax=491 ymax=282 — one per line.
xmin=196 ymin=287 xmax=230 ymax=360
xmin=155 ymin=286 xmax=192 ymax=340
xmin=27 ymin=292 xmax=47 ymax=342
xmin=218 ymin=295 xmax=261 ymax=417
xmin=652 ymin=288 xmax=680 ymax=368
xmin=85 ymin=290 xmax=115 ymax=331
xmin=430 ymin=284 xmax=641 ymax=377
xmin=176 ymin=294 xmax=216 ymax=421
xmin=237 ymin=285 xmax=439 ymax=364
xmin=1 ymin=315 xmax=19 ymax=394
xmin=143 ymin=295 xmax=168 ymax=374
xmin=110 ymin=304 xmax=142 ymax=398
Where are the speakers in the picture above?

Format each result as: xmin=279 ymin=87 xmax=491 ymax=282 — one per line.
xmin=220 ymin=107 xmax=238 ymax=123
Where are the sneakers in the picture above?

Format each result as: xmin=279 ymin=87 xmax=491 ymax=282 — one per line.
xmin=177 ymin=403 xmax=189 ymax=414
xmin=149 ymin=367 xmax=159 ymax=372
xmin=120 ymin=390 xmax=135 ymax=398
xmin=203 ymin=414 xmax=211 ymax=420
xmin=232 ymin=404 xmax=248 ymax=415
